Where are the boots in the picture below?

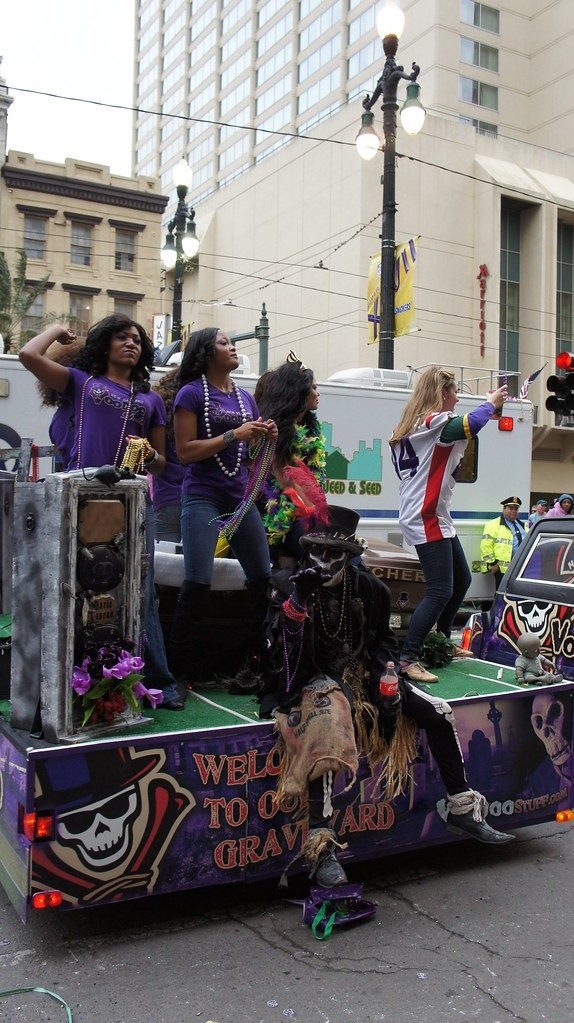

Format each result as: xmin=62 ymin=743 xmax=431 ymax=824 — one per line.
xmin=166 ymin=581 xmax=210 ymax=680
xmin=244 ymin=578 xmax=268 ymax=643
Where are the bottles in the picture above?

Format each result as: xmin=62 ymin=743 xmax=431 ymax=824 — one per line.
xmin=378 ymin=661 xmax=399 ymax=718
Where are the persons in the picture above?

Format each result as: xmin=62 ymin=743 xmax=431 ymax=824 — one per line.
xmin=479 ymin=496 xmax=527 ymax=595
xmin=515 ymin=632 xmax=564 ymax=685
xmin=528 ymin=493 xmax=574 ymax=529
xmin=388 ymin=365 xmax=508 ymax=684
xmin=172 ymin=327 xmax=279 ymax=692
xmin=255 ymin=502 xmax=516 ymax=888
xmin=16 ymin=312 xmax=186 ymax=711
xmin=149 ymin=365 xmax=186 ymax=542
xmin=249 ymin=361 xmax=366 ymax=566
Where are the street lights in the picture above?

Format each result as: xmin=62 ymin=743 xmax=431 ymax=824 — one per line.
xmin=158 ymin=156 xmax=202 ymax=353
xmin=352 ymin=1 xmax=426 ymax=363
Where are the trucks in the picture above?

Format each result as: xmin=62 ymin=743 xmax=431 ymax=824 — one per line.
xmin=0 ymin=352 xmax=534 ymax=632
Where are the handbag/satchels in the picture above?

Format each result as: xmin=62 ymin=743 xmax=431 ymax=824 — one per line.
xmin=303 ymin=882 xmax=376 ymax=940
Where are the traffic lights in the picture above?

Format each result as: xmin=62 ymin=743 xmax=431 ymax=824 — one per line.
xmin=544 ymin=350 xmax=574 ymax=416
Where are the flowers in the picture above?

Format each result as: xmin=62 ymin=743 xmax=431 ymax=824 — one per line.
xmin=69 ymin=636 xmax=164 ymax=729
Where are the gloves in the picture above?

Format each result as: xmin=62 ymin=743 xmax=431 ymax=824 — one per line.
xmin=290 ymin=566 xmax=330 ymax=612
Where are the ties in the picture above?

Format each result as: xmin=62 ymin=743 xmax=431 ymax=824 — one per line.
xmin=512 ymin=521 xmax=522 ymax=547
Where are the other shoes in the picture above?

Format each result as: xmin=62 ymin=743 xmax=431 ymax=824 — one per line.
xmin=403 ymin=662 xmax=439 ymax=682
xmin=447 ymin=810 xmax=516 ymax=843
xmin=162 ymin=688 xmax=185 ymax=711
xmin=450 ymin=643 xmax=473 ymax=657
xmin=313 ymin=841 xmax=348 ymax=888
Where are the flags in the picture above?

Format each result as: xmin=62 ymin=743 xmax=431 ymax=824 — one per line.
xmin=520 ymin=370 xmax=541 ymax=400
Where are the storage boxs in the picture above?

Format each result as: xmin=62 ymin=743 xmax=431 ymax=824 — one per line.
xmin=353 ymin=534 xmax=428 ymax=637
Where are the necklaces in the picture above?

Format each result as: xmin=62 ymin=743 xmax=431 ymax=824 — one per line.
xmin=77 ymin=375 xmax=134 ymax=469
xmin=200 ymin=372 xmax=247 ymax=476
xmin=206 ymin=423 xmax=277 ymax=541
xmin=314 ymin=567 xmax=359 ymax=672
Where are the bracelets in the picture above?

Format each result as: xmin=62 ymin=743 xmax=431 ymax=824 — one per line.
xmin=145 ymin=449 xmax=158 ymax=466
xmin=281 ymin=595 xmax=307 ymax=693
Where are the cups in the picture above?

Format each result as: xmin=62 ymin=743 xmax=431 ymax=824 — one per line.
xmin=489 ymin=390 xmax=503 ymax=419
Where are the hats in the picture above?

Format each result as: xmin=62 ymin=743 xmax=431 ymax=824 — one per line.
xmin=299 ymin=506 xmax=364 ymax=555
xmin=537 ymin=499 xmax=547 ymax=506
xmin=500 ymin=497 xmax=522 ymax=506
xmin=559 ymin=494 xmax=573 ymax=503
